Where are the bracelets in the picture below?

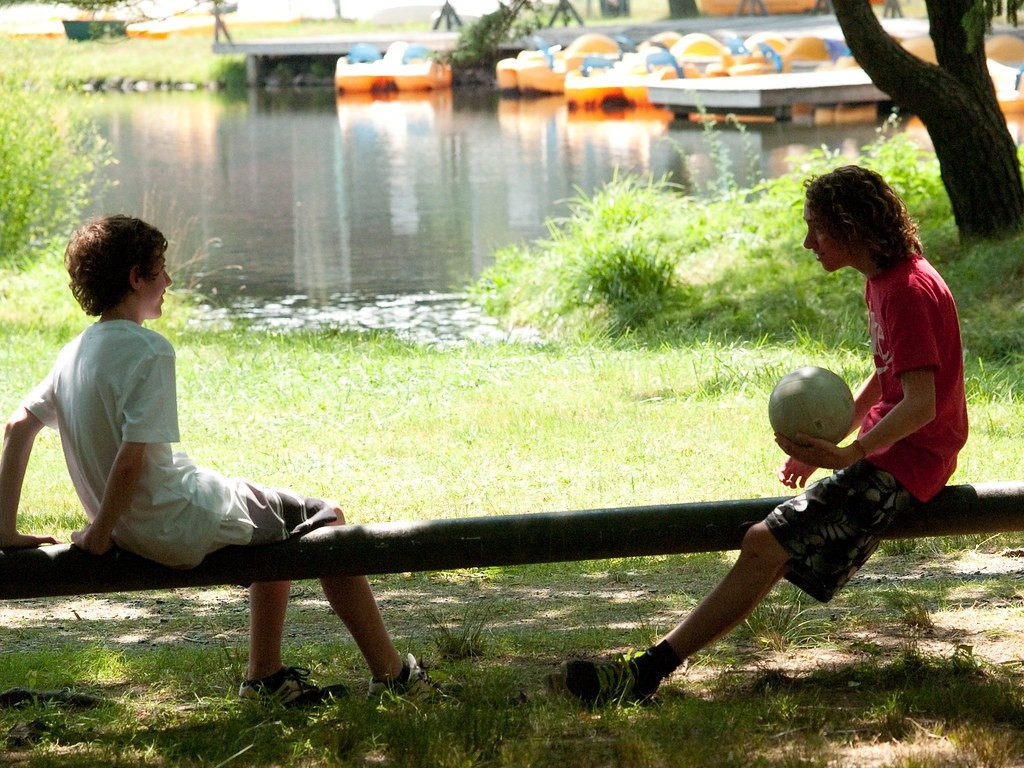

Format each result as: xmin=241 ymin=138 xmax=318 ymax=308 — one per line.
xmin=853 ymin=440 xmax=866 ymax=459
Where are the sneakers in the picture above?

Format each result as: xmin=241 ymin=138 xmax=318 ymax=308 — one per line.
xmin=238 ymin=654 xmax=463 ymax=711
xmin=551 ymin=648 xmax=663 ymax=709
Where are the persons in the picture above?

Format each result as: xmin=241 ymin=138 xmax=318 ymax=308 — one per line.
xmin=557 ymin=164 xmax=969 ymax=704
xmin=0 ymin=214 xmax=465 ymax=710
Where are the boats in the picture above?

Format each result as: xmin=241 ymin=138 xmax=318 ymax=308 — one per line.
xmin=335 ymin=20 xmax=1024 ymax=138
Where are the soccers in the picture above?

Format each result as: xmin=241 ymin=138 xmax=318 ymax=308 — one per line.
xmin=768 ymin=367 xmax=854 ymax=445
